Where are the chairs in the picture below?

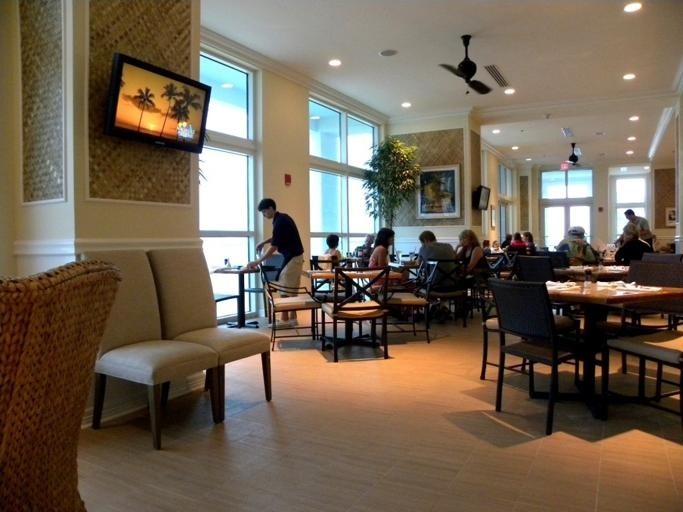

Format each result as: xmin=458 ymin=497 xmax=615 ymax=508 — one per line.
xmin=78 ymin=250 xmax=220 ymax=449
xmin=486 ymin=275 xmax=610 ymax=435
xmin=148 ymin=248 xmax=272 ymax=423
xmin=601 ymin=329 xmax=683 ymax=422
xmin=475 ymin=275 xmax=574 ymax=380
xmin=214 ymin=247 xmax=682 ymax=352
xmin=0 ymin=261 xmax=117 ymax=512
xmin=322 ymin=265 xmax=392 ymax=362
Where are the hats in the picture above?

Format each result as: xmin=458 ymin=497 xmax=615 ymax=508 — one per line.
xmin=567 ymin=226 xmax=586 ymax=242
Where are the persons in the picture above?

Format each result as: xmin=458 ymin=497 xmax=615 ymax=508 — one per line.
xmin=417 ymin=230 xmax=462 ymax=323
xmin=368 ymin=228 xmax=417 ymax=317
xmin=247 ymin=198 xmax=304 ymax=329
xmin=456 ymin=229 xmax=602 ymax=318
xmin=615 ymin=224 xmax=650 ymax=263
xmin=358 ymin=234 xmax=374 ymax=267
xmin=614 ymin=209 xmax=654 ymax=252
xmin=326 ymin=235 xmax=355 ymax=291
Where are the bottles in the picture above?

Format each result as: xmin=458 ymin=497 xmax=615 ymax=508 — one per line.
xmin=358 ymin=247 xmax=362 ymax=257
xmin=599 ymin=261 xmax=602 ymax=269
xmin=583 ymin=269 xmax=591 ymax=286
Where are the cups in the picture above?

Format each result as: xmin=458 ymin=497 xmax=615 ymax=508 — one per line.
xmin=395 ymin=250 xmax=401 ymax=263
xmin=408 ymin=250 xmax=415 ymax=262
xmin=606 ymin=243 xmax=614 ymax=257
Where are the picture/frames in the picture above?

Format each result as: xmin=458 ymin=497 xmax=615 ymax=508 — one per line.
xmin=415 ymin=164 xmax=461 ymax=219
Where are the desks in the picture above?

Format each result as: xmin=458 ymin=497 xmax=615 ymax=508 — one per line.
xmin=544 ymin=276 xmax=683 ymax=395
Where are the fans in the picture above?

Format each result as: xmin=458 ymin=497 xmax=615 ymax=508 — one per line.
xmin=438 ymin=35 xmax=492 ymax=94
xmin=564 ymin=143 xmax=582 ymax=167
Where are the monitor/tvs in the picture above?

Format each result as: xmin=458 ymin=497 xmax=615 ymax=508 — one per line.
xmin=99 ymin=53 xmax=211 ymax=153
xmin=473 ymin=185 xmax=490 ymax=213
